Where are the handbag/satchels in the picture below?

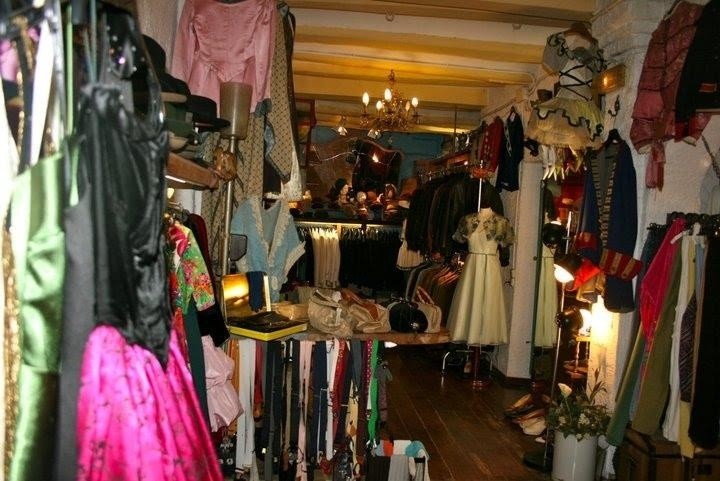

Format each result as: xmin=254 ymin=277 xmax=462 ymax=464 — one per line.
xmin=344 ymin=286 xmax=392 ymax=335
xmin=305 ymin=283 xmax=350 ymax=336
xmin=410 ymin=283 xmax=443 ymax=334
xmin=388 ymin=296 xmax=429 ymax=332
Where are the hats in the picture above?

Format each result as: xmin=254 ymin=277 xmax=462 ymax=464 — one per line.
xmin=187 ymin=92 xmax=230 ymax=133
xmin=139 ymin=30 xmax=189 ymax=95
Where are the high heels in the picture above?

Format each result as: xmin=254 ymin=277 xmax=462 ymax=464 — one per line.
xmin=504 ymin=393 xmax=536 ymax=418
xmin=514 ymin=409 xmax=547 ymax=435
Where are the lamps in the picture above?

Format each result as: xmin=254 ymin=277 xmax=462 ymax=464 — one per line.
xmin=522 ymin=206 xmax=584 ymax=474
xmin=593 ymin=61 xmax=625 ymax=94
xmin=356 ymin=68 xmax=425 ymax=146
xmin=334 ymin=112 xmax=350 ymax=138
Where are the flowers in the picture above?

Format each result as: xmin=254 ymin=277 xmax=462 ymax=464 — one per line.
xmin=542 ymin=344 xmax=614 ymax=440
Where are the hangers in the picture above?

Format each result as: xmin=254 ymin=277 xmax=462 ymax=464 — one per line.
xmin=561 ymin=20 xmax=600 ymax=46
xmin=599 ymin=113 xmax=624 ymax=159
xmin=643 ymin=210 xmax=719 ymax=243
xmin=481 ymin=113 xmax=487 ymax=126
xmin=506 ymin=101 xmax=517 ymax=121
xmin=493 ymin=107 xmax=501 ymax=121
xmin=430 ymin=256 xmax=468 ymax=290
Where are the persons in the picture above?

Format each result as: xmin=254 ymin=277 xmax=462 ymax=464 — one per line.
xmin=334 ymin=178 xmax=355 ymax=218
xmin=524 ymin=23 xmax=609 ymax=149
xmin=447 ymin=208 xmax=513 ymax=347
xmin=380 ymin=184 xmax=400 ymax=225
xmin=354 ymin=192 xmax=374 ymax=221
